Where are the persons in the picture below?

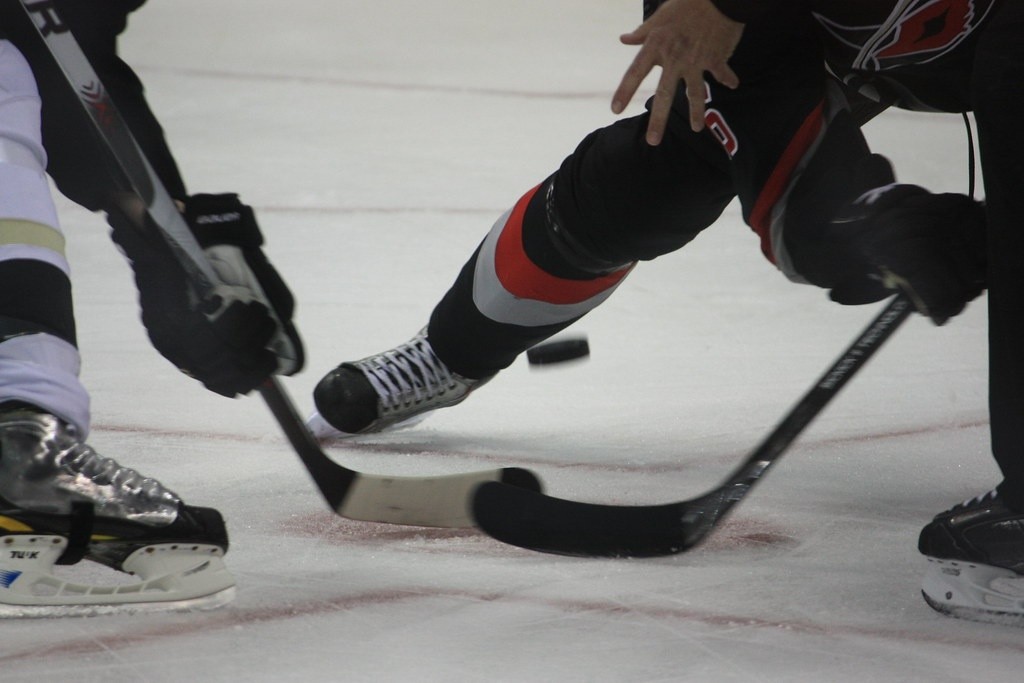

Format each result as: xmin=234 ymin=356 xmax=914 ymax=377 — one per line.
xmin=311 ymin=3 xmax=1023 ymax=628
xmin=3 ymin=0 xmax=308 ymax=619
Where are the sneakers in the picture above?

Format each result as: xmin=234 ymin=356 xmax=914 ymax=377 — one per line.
xmin=0 ymin=409 xmax=237 ymax=619
xmin=308 ymin=324 xmax=480 ymax=441
xmin=917 ymin=481 xmax=1024 ymax=628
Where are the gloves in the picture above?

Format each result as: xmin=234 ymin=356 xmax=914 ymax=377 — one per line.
xmin=112 ymin=192 xmax=305 ymax=398
xmin=826 ymin=183 xmax=989 ymax=326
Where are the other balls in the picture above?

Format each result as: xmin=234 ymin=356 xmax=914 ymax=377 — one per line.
xmin=527 ymin=333 xmax=591 ymax=369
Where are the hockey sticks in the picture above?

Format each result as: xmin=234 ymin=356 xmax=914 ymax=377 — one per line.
xmin=468 ymin=288 xmax=913 ymax=562
xmin=23 ymin=2 xmax=544 ymax=530
